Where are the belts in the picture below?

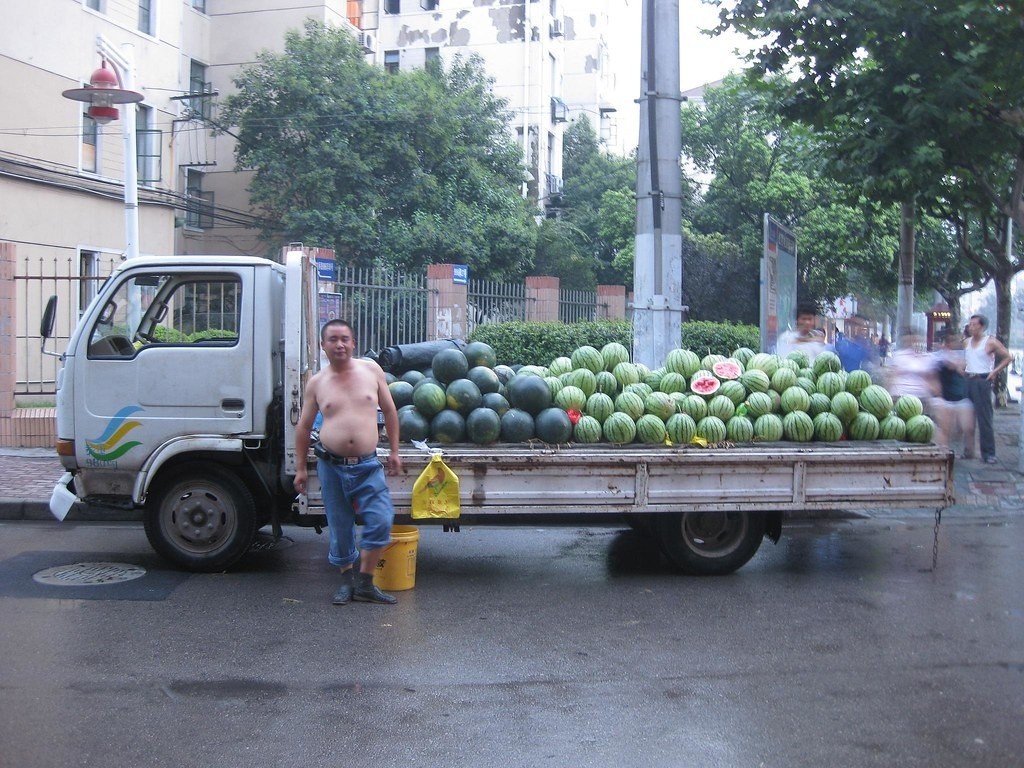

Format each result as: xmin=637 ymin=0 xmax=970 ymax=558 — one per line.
xmin=329 ymin=453 xmax=376 ymax=466
xmin=968 ymin=374 xmax=987 ymax=379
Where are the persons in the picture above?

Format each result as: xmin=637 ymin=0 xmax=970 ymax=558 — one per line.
xmin=293 ymin=319 xmax=400 ymax=605
xmin=777 ymin=302 xmax=977 ymax=461
xmin=955 ymin=314 xmax=1014 ymax=464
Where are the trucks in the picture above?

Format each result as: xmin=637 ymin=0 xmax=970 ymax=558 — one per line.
xmin=38 ymin=242 xmax=955 ymax=573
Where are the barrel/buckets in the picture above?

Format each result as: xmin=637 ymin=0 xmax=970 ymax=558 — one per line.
xmin=373 ymin=525 xmax=419 ymax=590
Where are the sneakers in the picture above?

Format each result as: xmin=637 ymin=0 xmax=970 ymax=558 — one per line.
xmin=332 ymin=584 xmax=353 ymax=604
xmin=352 ymin=586 xmax=396 ymax=603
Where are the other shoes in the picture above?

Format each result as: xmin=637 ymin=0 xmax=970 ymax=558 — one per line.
xmin=985 ymin=456 xmax=996 ymax=464
xmin=956 ymin=453 xmax=974 ymax=460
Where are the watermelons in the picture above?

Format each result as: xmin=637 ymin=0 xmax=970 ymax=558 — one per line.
xmin=382 ymin=342 xmax=934 ymax=444
